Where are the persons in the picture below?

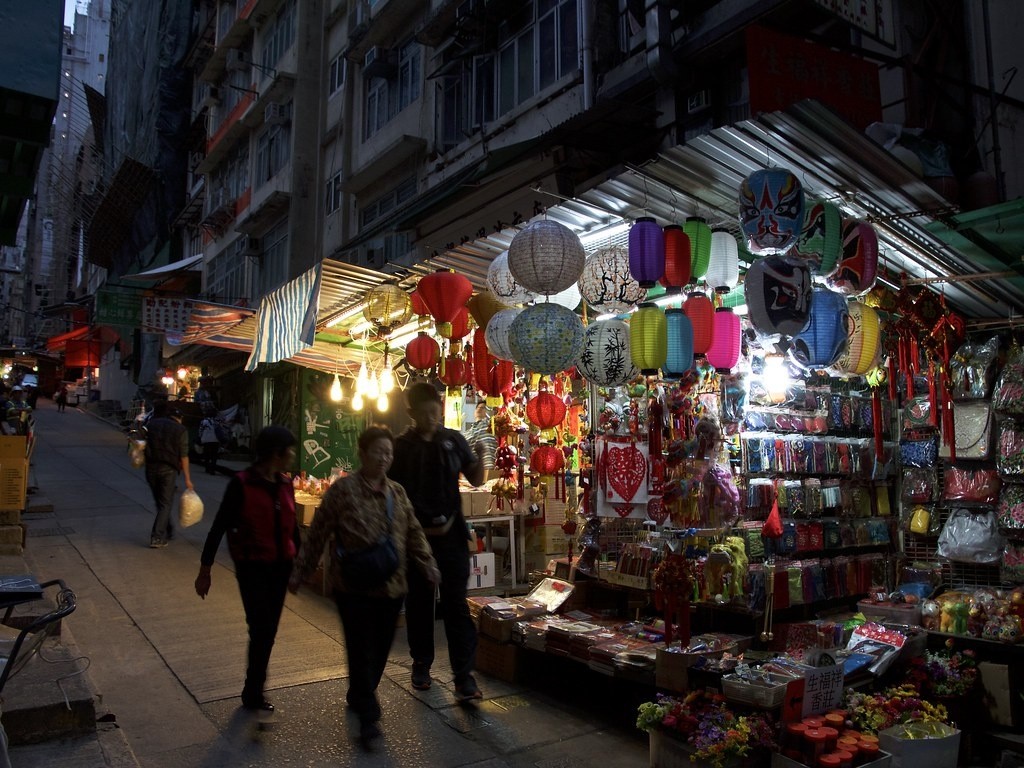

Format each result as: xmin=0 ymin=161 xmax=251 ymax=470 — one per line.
xmin=385 ymin=383 xmax=486 ymax=709
xmin=196 ymin=379 xmax=217 ymax=411
xmin=58 ymin=384 xmax=68 ymax=414
xmin=176 ymin=379 xmax=187 ymax=401
xmin=25 ymin=384 xmax=38 ymax=409
xmin=288 ymin=427 xmax=440 ymax=751
xmin=0 ymin=385 xmax=32 ymax=435
xmin=195 ymin=427 xmax=300 ymax=711
xmin=143 ymin=408 xmax=193 ymax=548
xmin=465 ymin=400 xmax=501 ymax=484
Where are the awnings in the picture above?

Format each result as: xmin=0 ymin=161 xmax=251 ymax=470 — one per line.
xmin=45 ymin=326 xmax=92 ymax=353
xmin=244 ymin=258 xmax=399 ymax=372
xmin=139 ymin=297 xmax=361 ymax=379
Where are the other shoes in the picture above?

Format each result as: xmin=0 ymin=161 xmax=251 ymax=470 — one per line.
xmin=410 ymin=658 xmax=432 ymax=690
xmin=150 ymin=536 xmax=168 ymax=547
xmin=241 ymin=688 xmax=275 ymax=713
xmin=453 ymin=671 xmax=484 ymax=699
xmin=168 ymin=533 xmax=180 ymax=540
xmin=347 ymin=686 xmax=381 ymax=737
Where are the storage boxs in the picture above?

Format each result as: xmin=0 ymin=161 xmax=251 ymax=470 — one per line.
xmin=480 ymin=608 xmax=545 ymax=642
xmin=772 ymin=746 xmax=893 ymax=768
xmin=460 ymin=474 xmax=580 ymax=591
xmin=475 ymin=636 xmax=533 ymax=683
xmin=0 ymin=434 xmax=37 ymax=511
xmin=721 ymin=670 xmax=798 ymax=708
xmin=293 ymin=496 xmax=322 ymax=525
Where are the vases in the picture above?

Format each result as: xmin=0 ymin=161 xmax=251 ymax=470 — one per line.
xmin=640 ymin=725 xmax=744 ymax=768
xmin=932 ymin=695 xmax=968 ymax=721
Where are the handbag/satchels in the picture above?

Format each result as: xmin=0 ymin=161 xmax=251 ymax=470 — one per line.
xmin=0 ymin=575 xmax=43 ymax=609
xmin=179 ymin=484 xmax=203 ymax=526
xmin=348 ymin=539 xmax=398 ymax=580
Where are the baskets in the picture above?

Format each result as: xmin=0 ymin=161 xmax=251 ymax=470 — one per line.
xmin=721 ymin=673 xmax=786 ymax=707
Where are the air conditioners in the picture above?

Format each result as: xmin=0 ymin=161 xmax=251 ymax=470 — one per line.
xmin=238 ymin=238 xmax=264 ymax=256
xmin=264 ymin=101 xmax=290 ymax=126
xmin=365 ymin=46 xmax=392 ymax=65
xmin=207 ymin=187 xmax=231 ymax=215
xmin=203 ymin=86 xmax=222 ymax=106
xmin=226 ymin=48 xmax=249 ymax=70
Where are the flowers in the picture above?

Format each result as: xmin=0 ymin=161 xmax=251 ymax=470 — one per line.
xmin=638 ymin=690 xmax=779 ymax=768
xmin=904 ymin=638 xmax=977 ymax=699
xmin=845 ymin=683 xmax=947 ymax=734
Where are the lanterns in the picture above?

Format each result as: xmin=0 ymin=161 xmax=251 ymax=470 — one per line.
xmin=485 ymin=216 xmax=647 ymax=387
xmin=406 ymin=267 xmax=514 ymax=406
xmin=629 ymin=215 xmax=741 ymax=380
xmin=364 ymin=283 xmax=413 ymax=340
xmin=526 ymin=394 xmax=567 ymax=440
xmin=739 ymin=166 xmax=880 ymax=376
xmin=531 ymin=445 xmax=564 ymax=486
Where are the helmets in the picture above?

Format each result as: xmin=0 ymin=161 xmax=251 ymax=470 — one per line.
xmin=11 ymin=386 xmax=23 ymax=394
